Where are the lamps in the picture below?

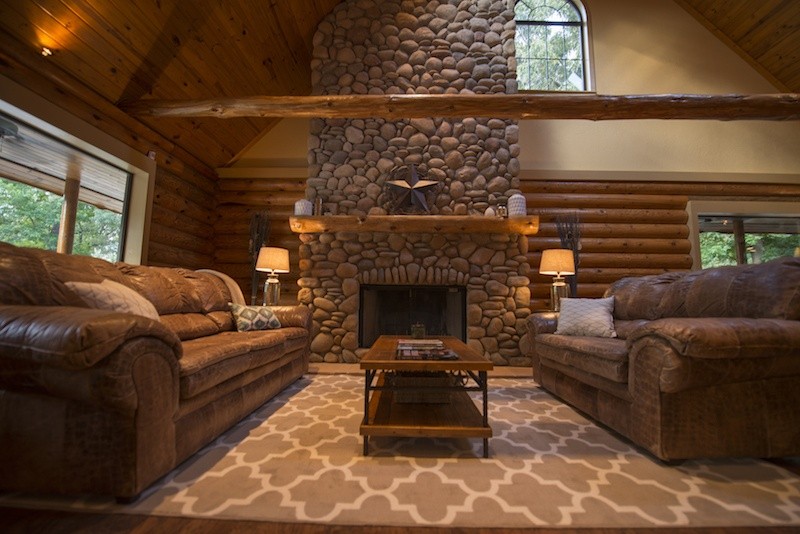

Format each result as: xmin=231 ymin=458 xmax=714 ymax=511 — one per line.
xmin=255 ymin=245 xmax=290 ymax=306
xmin=538 ymin=249 xmax=576 ymax=312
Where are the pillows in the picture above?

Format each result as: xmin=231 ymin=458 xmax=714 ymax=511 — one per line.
xmin=65 ymin=278 xmax=160 ymax=322
xmin=555 ymin=294 xmax=616 ymax=337
xmin=227 ymin=302 xmax=282 ymax=333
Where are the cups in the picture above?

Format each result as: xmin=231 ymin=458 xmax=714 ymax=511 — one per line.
xmin=314 ymin=197 xmax=322 ymax=216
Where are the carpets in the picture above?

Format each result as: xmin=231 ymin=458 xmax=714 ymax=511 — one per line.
xmin=1 ymin=371 xmax=799 ymax=526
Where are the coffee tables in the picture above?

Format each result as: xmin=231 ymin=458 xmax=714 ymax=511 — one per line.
xmin=359 ymin=332 xmax=494 ymax=460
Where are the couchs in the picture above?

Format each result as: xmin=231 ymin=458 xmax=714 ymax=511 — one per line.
xmin=0 ymin=241 xmax=312 ymax=504
xmin=524 ymin=255 xmax=800 ymax=464
